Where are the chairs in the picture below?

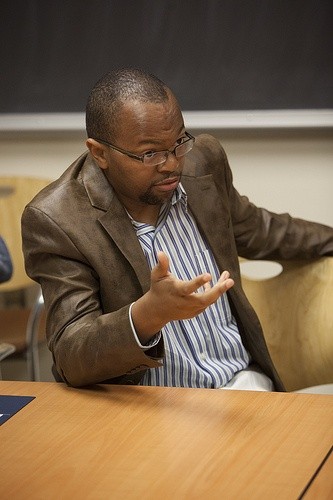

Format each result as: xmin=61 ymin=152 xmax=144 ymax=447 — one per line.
xmin=238 ymin=257 xmax=333 ymax=390
xmin=0 ymin=175 xmax=54 ymax=381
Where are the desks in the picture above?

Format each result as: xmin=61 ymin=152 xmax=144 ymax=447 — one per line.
xmin=0 ymin=381 xmax=333 ymax=500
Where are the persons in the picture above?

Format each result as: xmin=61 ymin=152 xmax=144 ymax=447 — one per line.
xmin=20 ymin=67 xmax=333 ymax=393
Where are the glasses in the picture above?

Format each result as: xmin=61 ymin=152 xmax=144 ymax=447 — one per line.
xmin=91 ymin=131 xmax=196 ymax=167
